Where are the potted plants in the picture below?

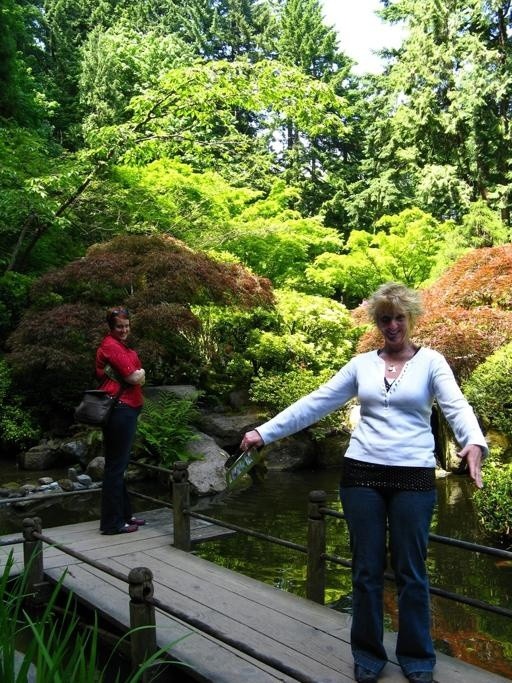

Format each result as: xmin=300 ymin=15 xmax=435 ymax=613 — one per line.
xmin=8 ymin=232 xmax=275 ymax=426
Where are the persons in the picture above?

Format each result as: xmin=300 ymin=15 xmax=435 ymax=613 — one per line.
xmin=94 ymin=304 xmax=149 ymax=538
xmin=238 ymin=279 xmax=490 ymax=682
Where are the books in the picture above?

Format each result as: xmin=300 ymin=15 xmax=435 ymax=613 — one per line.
xmin=224 ymin=445 xmax=262 ymax=492
xmin=103 ymin=362 xmax=132 ymax=389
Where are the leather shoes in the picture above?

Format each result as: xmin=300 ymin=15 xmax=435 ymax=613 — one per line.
xmin=408 ymin=671 xmax=433 ymax=683
xmin=128 ymin=517 xmax=146 ymax=526
xmin=353 ymin=661 xmax=378 ymax=683
xmin=121 ymin=521 xmax=138 ymax=533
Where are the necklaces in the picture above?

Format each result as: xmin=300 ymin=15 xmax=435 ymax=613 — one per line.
xmin=388 ymin=362 xmax=400 ymax=373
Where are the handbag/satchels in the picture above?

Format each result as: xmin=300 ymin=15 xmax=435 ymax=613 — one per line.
xmin=72 ymin=386 xmax=119 ymax=428
